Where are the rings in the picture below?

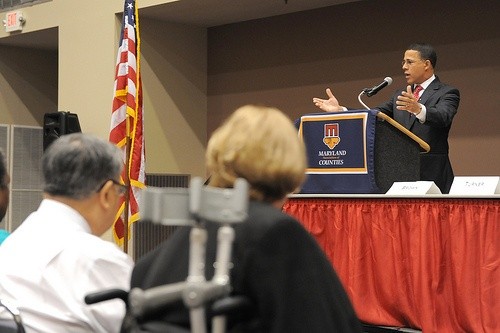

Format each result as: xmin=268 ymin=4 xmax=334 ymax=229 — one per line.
xmin=407 ymin=102 xmax=410 ymax=106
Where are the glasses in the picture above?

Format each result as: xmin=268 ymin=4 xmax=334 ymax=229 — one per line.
xmin=402 ymin=59 xmax=414 ymax=65
xmin=95 ymin=179 xmax=128 ymax=195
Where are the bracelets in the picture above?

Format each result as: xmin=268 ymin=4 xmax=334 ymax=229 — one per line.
xmin=416 ymin=108 xmax=422 ymax=116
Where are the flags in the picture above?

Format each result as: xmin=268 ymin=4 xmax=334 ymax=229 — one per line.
xmin=109 ymin=0 xmax=145 ymax=246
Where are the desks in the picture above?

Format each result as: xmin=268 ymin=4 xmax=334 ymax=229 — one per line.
xmin=284 ymin=194 xmax=500 ymax=333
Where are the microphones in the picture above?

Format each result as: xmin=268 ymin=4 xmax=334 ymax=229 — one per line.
xmin=366 ymin=77 xmax=392 ymax=97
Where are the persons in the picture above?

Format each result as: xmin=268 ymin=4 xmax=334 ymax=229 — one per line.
xmin=313 ymin=43 xmax=460 ymax=194
xmin=0 ymin=134 xmax=134 ymax=333
xmin=121 ymin=105 xmax=364 ymax=333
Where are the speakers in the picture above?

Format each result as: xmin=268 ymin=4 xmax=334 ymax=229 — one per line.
xmin=42 ymin=112 xmax=82 ymax=153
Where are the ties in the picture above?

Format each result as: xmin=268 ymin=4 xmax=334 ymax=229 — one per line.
xmin=412 ymin=85 xmax=423 ymax=101
